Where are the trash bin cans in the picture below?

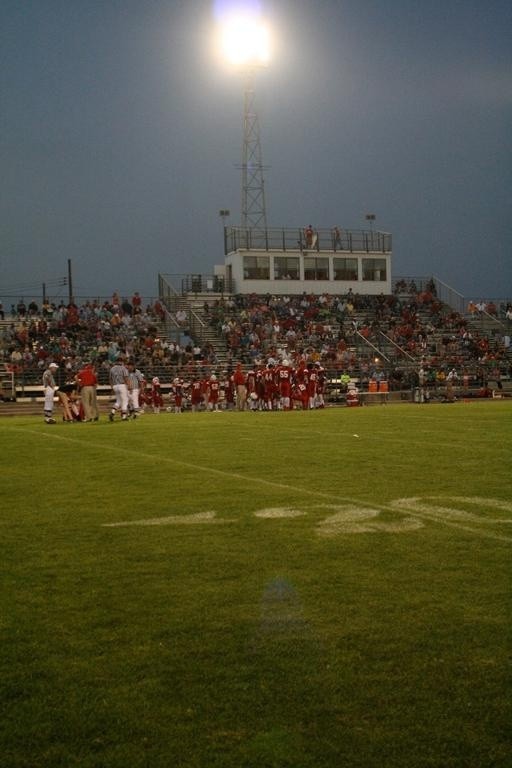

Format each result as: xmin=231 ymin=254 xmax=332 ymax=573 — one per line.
xmin=413 ymin=387 xmax=420 ymax=402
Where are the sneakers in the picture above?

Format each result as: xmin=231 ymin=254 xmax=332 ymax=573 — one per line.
xmin=42 ymin=407 xmax=140 ymax=425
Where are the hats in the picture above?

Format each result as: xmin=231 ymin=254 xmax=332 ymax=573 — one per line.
xmin=48 ymin=362 xmax=60 ymax=369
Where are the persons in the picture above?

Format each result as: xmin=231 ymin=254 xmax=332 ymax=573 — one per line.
xmin=305 ymin=224 xmax=313 ymax=248
xmin=1 ymin=269 xmax=512 ymax=424
xmin=333 ymin=225 xmax=344 ymax=250
